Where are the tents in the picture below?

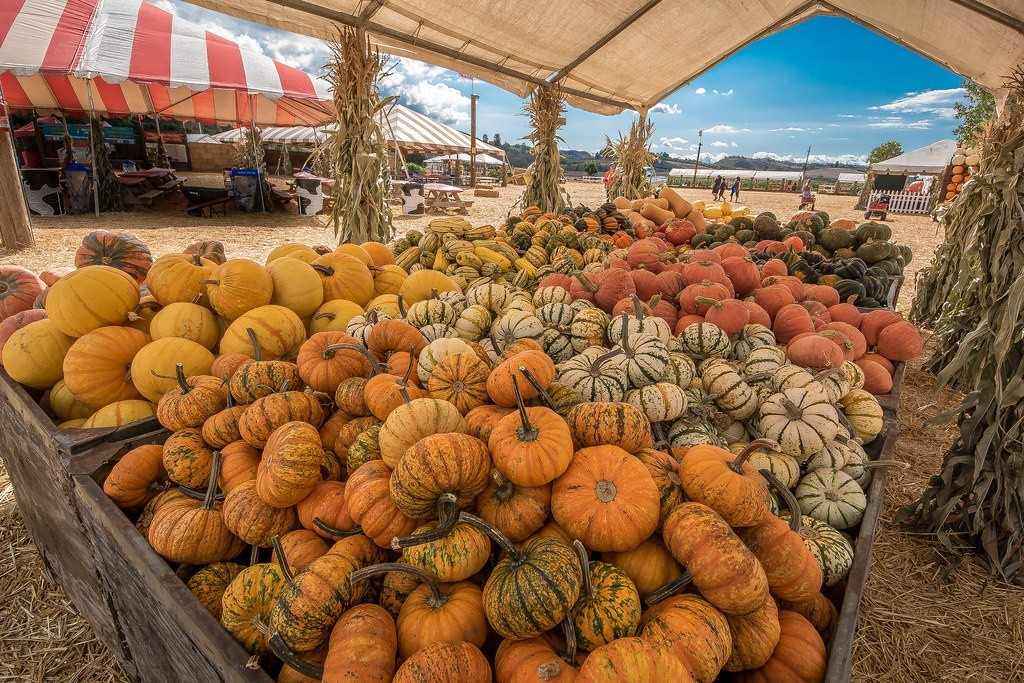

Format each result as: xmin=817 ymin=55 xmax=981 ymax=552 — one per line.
xmin=214 ymin=91 xmax=507 ymax=186
xmin=423 ymin=153 xmax=508 ymax=174
xmin=0 ymin=1 xmax=336 ymax=214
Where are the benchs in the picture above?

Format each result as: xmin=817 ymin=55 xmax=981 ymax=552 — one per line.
xmin=186 ymin=196 xmax=231 ymax=219
xmin=138 ymin=175 xmax=189 ymax=207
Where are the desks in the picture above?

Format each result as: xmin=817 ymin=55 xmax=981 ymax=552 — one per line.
xmin=424 ymin=183 xmax=474 ymax=214
xmin=117 ymin=165 xmax=184 ymax=201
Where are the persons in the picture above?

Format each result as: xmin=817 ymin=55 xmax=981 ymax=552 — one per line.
xmin=603 ymin=163 xmax=615 ymax=203
xmin=712 ymin=175 xmax=741 ymax=202
xmin=799 ymin=179 xmax=812 ymax=206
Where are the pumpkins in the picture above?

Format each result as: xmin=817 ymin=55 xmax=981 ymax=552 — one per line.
xmin=945 ymin=147 xmax=978 ymax=200
xmin=0 ymin=187 xmax=922 ymax=683
